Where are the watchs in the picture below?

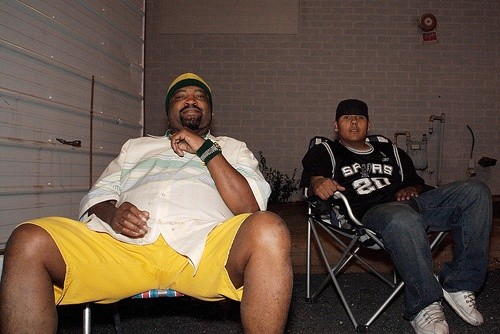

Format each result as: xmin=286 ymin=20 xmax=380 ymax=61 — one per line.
xmin=201 ymin=143 xmax=221 ymax=161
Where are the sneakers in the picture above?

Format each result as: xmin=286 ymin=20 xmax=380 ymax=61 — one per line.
xmin=409 ymin=301 xmax=449 ymax=334
xmin=436 ymin=275 xmax=484 ymax=326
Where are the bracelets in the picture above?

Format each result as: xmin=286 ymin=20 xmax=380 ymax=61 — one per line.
xmin=195 ymin=139 xmax=220 ymax=163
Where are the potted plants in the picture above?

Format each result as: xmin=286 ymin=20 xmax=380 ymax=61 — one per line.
xmin=258 ymin=151 xmax=300 ymax=214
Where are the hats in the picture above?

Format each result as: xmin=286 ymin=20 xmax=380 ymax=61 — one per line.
xmin=336 ymin=99 xmax=368 ymax=121
xmin=165 ymin=73 xmax=213 ymax=112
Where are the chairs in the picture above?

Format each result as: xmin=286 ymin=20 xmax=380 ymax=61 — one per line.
xmin=303 ymin=136 xmax=450 ymax=334
xmin=82 ymin=288 xmax=186 ymax=334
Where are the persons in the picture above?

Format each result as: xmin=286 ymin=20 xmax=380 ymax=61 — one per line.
xmin=0 ymin=73 xmax=294 ymax=334
xmin=300 ymin=99 xmax=494 ymax=334
